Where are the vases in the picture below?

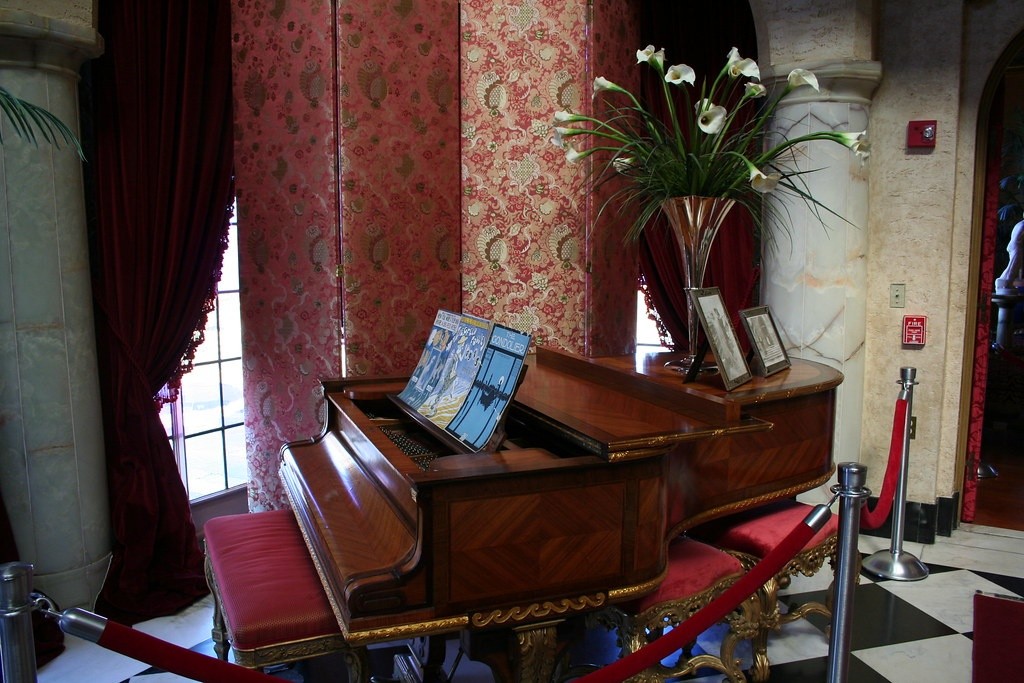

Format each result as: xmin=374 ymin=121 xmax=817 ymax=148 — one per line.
xmin=660 ymin=197 xmax=738 ymax=374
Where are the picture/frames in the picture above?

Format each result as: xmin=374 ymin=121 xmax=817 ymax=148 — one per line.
xmin=737 ymin=306 xmax=792 ymax=377
xmin=686 ymin=288 xmax=753 ymax=390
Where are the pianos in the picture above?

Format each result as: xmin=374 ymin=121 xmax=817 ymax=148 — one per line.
xmin=275 ymin=348 xmax=844 ymax=683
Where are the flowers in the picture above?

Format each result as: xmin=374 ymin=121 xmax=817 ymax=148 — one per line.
xmin=550 ymin=42 xmax=870 ymax=257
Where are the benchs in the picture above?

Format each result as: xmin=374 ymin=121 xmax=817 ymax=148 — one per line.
xmin=202 ymin=510 xmax=369 ymax=683
xmin=596 ymin=500 xmax=864 ymax=683
